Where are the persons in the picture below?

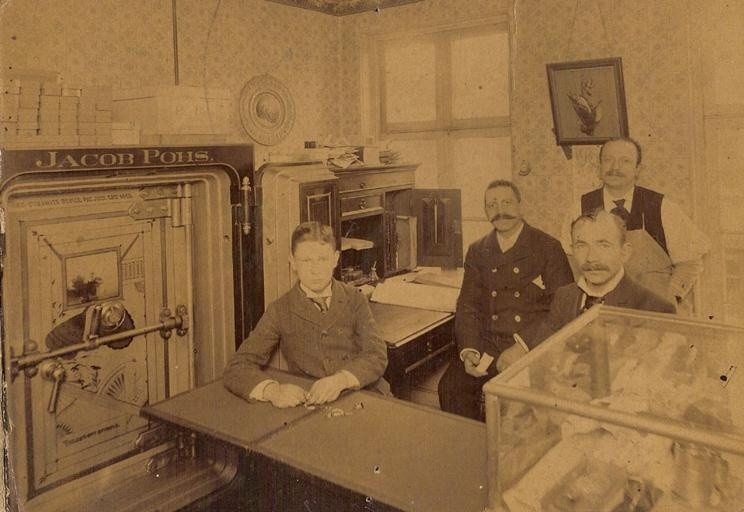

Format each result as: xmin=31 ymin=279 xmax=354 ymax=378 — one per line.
xmin=496 ymin=207 xmax=677 ymax=374
xmin=222 ymin=220 xmax=396 ymax=511
xmin=437 ymin=178 xmax=575 ymax=425
xmin=567 ymin=136 xmax=705 ymax=317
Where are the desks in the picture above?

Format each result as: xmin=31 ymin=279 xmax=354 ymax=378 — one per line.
xmin=141 ymin=360 xmax=491 ymax=510
xmin=368 ymin=300 xmax=456 ymax=389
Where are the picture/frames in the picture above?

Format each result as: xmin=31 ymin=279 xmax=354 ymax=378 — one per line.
xmin=546 ymin=56 xmax=629 ymax=149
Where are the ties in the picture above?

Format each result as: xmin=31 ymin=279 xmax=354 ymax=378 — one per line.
xmin=584 ymin=295 xmax=603 ymax=310
xmin=613 ymin=199 xmax=629 ymax=218
xmin=310 ymin=296 xmax=327 ymax=313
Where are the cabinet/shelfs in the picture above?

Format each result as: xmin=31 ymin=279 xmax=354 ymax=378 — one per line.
xmin=297 ymin=160 xmax=464 ymax=289
